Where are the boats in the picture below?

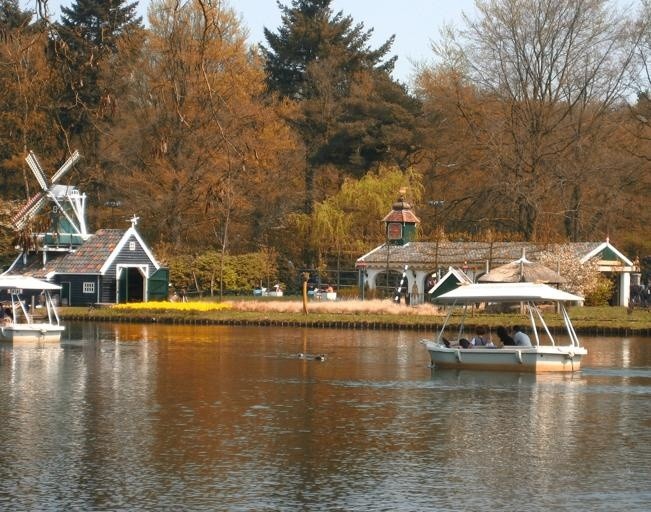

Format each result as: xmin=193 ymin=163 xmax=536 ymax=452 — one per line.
xmin=0 ymin=275 xmax=65 ymax=345
xmin=420 ymin=282 xmax=587 ymax=374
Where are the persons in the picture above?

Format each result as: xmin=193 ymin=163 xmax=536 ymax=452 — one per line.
xmin=2 ymin=307 xmax=13 ymax=323
xmin=513 ymin=324 xmax=532 ymax=346
xmin=497 ymin=326 xmax=515 ymax=348
xmin=471 ymin=325 xmax=489 ymax=345
